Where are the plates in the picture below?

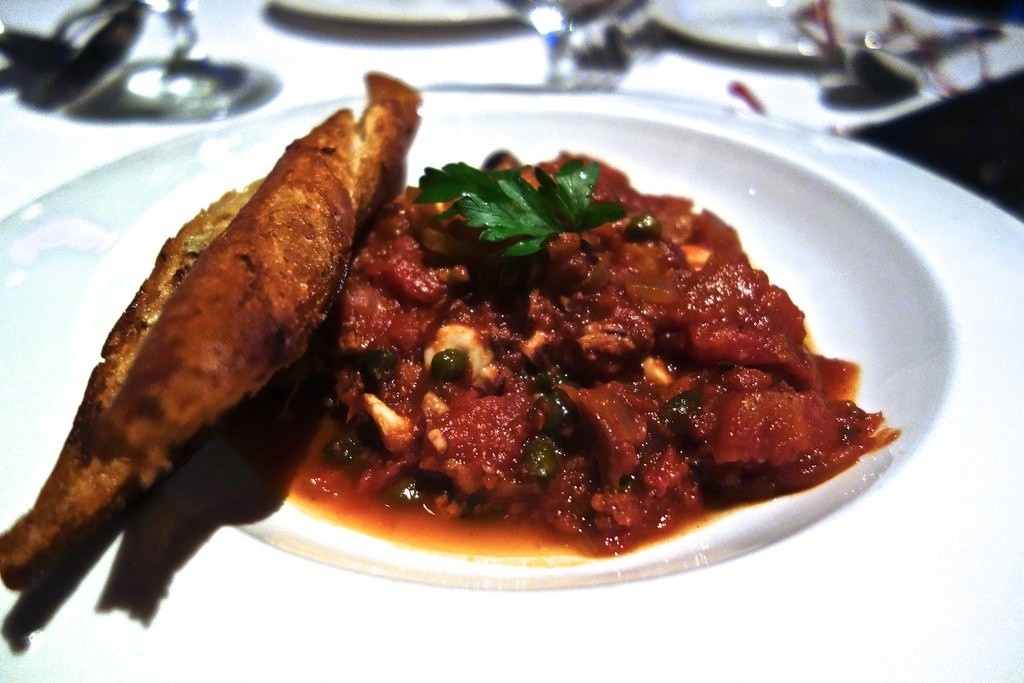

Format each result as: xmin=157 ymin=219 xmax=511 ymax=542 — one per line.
xmin=261 ymin=1 xmax=525 ymax=32
xmin=0 ymin=84 xmax=1024 ymax=683
xmin=647 ymin=1 xmax=943 ymax=64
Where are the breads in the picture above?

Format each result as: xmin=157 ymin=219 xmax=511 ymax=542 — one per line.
xmin=0 ymin=70 xmax=420 ymax=592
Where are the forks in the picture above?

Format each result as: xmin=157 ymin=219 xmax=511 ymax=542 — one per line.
xmin=568 ymin=15 xmax=653 ymax=68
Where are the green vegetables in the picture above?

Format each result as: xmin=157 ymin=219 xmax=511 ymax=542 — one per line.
xmin=412 ymin=158 xmax=627 ymax=258
